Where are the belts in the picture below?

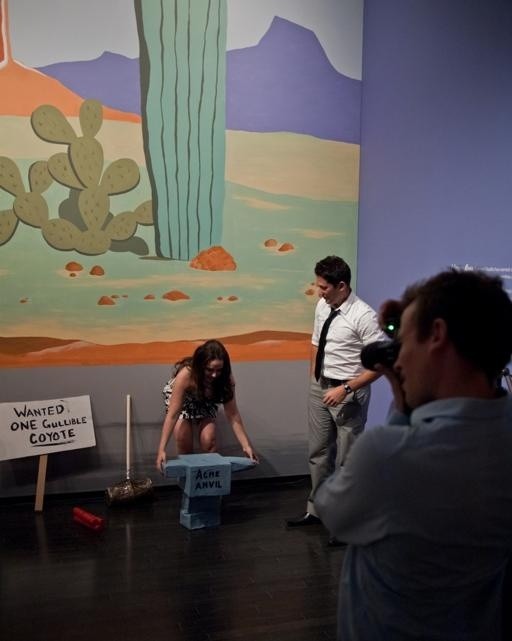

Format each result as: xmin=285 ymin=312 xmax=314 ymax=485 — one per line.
xmin=321 ymin=376 xmax=343 ymax=386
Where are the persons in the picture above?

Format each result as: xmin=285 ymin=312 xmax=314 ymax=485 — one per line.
xmin=155 ymin=338 xmax=259 ymax=476
xmin=283 ymin=255 xmax=392 ymax=546
xmin=314 ymin=267 xmax=512 ymax=641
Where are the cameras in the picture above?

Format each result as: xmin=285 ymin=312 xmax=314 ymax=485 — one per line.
xmin=361 ymin=339 xmax=401 ymax=372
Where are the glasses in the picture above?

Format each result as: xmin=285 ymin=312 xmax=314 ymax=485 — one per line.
xmin=314 ymin=307 xmax=339 ymax=382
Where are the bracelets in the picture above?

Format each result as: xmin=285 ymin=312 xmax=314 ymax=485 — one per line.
xmin=343 ymin=382 xmax=352 ymax=394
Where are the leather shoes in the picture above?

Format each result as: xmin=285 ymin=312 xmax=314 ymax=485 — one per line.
xmin=327 ymin=536 xmax=348 ymax=547
xmin=287 ymin=512 xmax=317 ymax=525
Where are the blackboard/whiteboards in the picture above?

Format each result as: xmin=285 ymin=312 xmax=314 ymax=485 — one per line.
xmin=0 ymin=394 xmax=96 ymax=462
